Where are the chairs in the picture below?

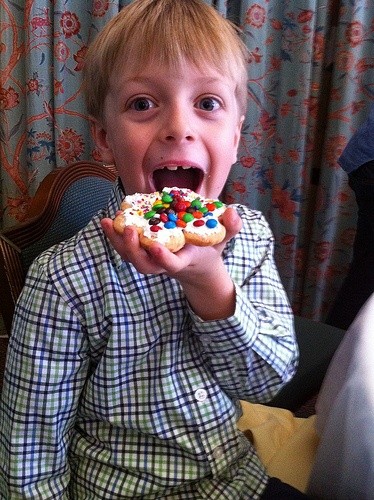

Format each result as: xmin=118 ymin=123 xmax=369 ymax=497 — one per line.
xmin=0 ymin=160 xmax=119 ymax=339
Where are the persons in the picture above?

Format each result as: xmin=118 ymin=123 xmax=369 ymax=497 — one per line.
xmin=0 ymin=1 xmax=310 ymax=500
xmin=338 ymin=114 xmax=374 ymax=329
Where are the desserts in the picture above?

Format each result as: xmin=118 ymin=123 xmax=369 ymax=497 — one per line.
xmin=115 ymin=186 xmax=228 ymax=254
